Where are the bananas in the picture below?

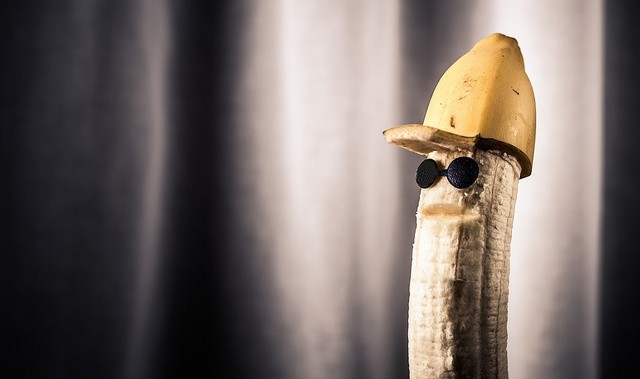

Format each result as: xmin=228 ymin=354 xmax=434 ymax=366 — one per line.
xmin=383 ymin=32 xmax=536 ymax=378
